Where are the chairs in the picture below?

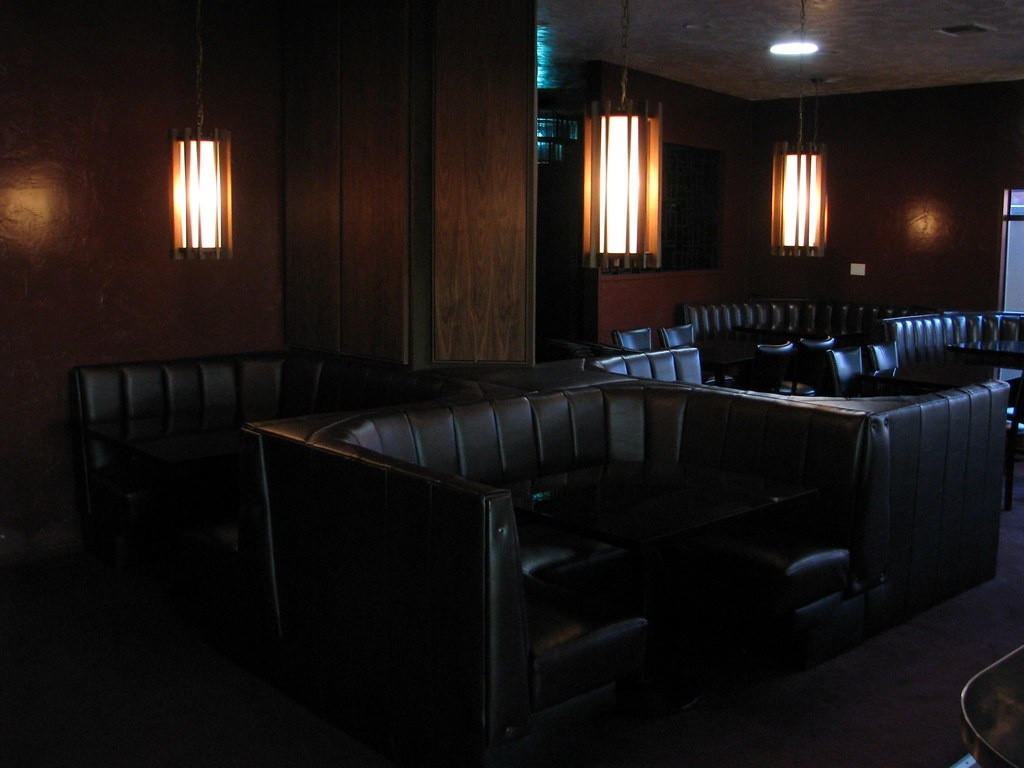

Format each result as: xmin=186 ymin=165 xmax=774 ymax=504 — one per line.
xmin=826 ymin=345 xmax=864 ymax=398
xmin=773 ymin=335 xmax=836 ymax=397
xmin=611 ymin=326 xmax=653 ymax=353
xmin=749 ymin=340 xmax=794 ymax=394
xmin=658 ymin=323 xmax=696 ymax=349
xmin=868 ymin=340 xmax=899 ymax=369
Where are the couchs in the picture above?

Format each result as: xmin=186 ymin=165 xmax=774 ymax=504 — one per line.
xmin=65 ymin=294 xmax=1024 ymax=768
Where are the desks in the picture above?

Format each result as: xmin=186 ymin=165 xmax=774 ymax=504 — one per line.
xmin=86 ymin=402 xmax=313 ymax=619
xmin=870 ymin=360 xmax=1024 ymax=407
xmin=681 ymin=339 xmax=769 ymax=388
xmin=737 ymin=320 xmax=878 ymax=344
xmin=950 ymin=340 xmax=1024 ymax=369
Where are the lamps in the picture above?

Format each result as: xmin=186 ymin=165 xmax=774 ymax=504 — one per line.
xmin=771 ymin=0 xmax=827 ymax=258
xmin=582 ymin=0 xmax=663 ymax=274
xmin=907 ymin=197 xmax=939 ymax=237
xmin=167 ymin=0 xmax=233 ymax=259
xmin=806 ymin=78 xmax=826 ymax=256
